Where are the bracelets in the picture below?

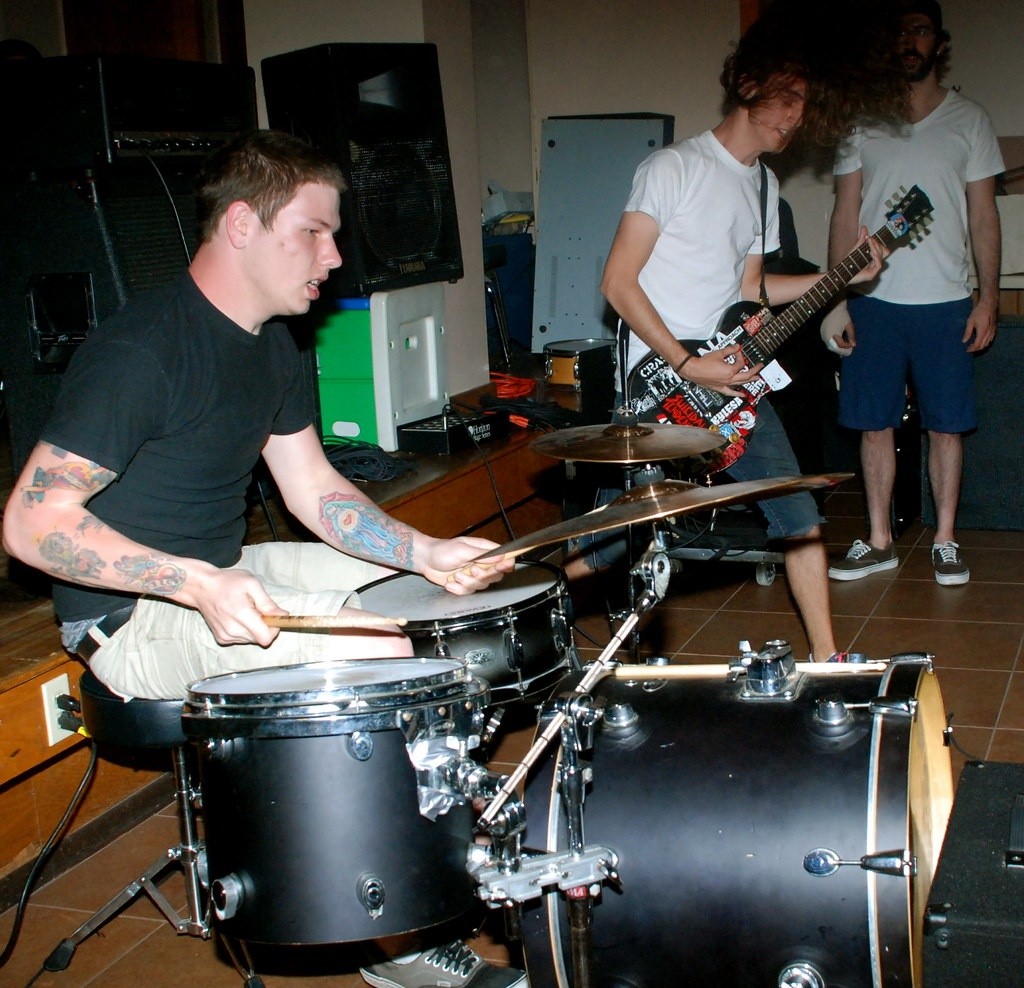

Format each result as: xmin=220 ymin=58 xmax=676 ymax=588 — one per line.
xmin=674 ymin=354 xmax=693 ymax=374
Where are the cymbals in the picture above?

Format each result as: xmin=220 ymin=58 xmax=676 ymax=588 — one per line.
xmin=528 ymin=422 xmax=728 ymax=464
xmin=463 ymin=471 xmax=856 ymax=565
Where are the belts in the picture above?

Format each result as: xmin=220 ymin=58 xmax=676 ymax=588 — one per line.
xmin=76 ymin=603 xmax=136 ymax=662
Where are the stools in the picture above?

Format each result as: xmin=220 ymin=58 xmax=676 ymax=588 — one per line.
xmin=483 ymin=244 xmax=513 ymax=369
xmin=43 ymin=670 xmax=266 ymax=988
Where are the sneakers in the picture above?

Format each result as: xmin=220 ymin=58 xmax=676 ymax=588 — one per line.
xmin=808 ymin=653 xmax=868 ymax=663
xmin=359 ymin=938 xmax=529 ymax=988
xmin=828 ymin=540 xmax=899 ymax=580
xmin=931 ymin=540 xmax=970 ymax=585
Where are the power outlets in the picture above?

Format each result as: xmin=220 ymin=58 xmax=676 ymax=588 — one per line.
xmin=40 ymin=672 xmax=74 ymax=747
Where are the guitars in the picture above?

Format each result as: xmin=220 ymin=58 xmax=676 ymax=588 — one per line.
xmin=622 ymin=182 xmax=936 ymax=478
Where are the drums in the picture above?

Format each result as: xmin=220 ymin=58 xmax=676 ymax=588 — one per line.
xmin=180 ymin=654 xmax=494 ymax=948
xmin=542 ymin=337 xmax=617 ymax=391
xmin=514 ymin=650 xmax=957 ymax=988
xmin=352 ymin=558 xmax=575 ymax=707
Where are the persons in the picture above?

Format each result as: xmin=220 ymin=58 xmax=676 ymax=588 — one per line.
xmin=820 ymin=0 xmax=1005 ymax=589
xmin=525 ymin=33 xmax=891 ymax=666
xmin=0 ymin=127 xmax=536 ymax=987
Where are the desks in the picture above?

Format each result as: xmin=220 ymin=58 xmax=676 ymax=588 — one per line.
xmin=919 ymin=315 xmax=1024 ymax=532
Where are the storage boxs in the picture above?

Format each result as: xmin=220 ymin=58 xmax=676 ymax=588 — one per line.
xmin=314 ymin=281 xmax=451 ymax=452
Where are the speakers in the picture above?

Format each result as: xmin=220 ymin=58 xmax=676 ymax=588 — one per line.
xmin=921 ymin=314 xmax=1024 ymax=532
xmin=261 ymin=42 xmax=464 ymax=302
xmin=0 ymin=155 xmax=323 ymax=600
